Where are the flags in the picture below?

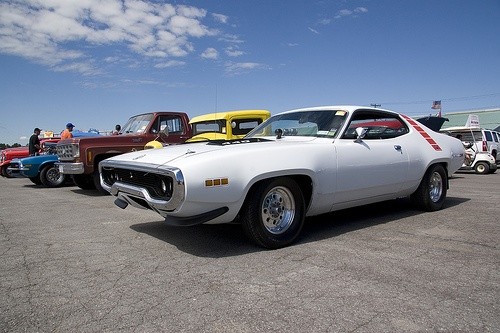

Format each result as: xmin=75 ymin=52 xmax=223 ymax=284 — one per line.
xmin=431 ymin=101 xmax=441 ymax=109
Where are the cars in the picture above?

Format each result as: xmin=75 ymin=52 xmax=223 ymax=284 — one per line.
xmin=344 ymin=114 xmax=500 ymax=175
xmin=0 ymin=129 xmax=105 ymax=188
xmin=54 ymin=111 xmax=193 ymax=193
xmin=98 ymin=105 xmax=466 ymax=250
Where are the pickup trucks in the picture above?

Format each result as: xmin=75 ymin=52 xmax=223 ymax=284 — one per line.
xmin=144 ymin=110 xmax=336 ymax=150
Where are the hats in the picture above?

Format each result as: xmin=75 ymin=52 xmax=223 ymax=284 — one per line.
xmin=34 ymin=128 xmax=42 ymax=132
xmin=66 ymin=123 xmax=76 ymax=127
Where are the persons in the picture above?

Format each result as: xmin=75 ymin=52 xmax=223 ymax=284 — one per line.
xmin=113 ymin=125 xmax=121 ymax=135
xmin=28 ymin=128 xmax=42 ymax=156
xmin=455 ymin=134 xmax=474 ymax=166
xmin=60 ymin=123 xmax=75 ymax=139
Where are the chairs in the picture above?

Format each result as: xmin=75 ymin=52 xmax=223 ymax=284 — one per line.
xmin=160 ymin=125 xmax=168 ymax=131
xmin=456 ymin=134 xmax=462 ymax=139
xmin=137 ymin=126 xmax=147 ymax=133
